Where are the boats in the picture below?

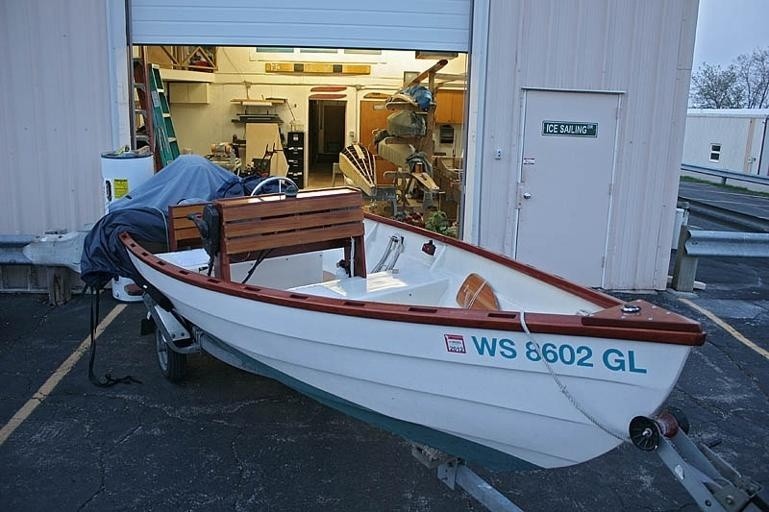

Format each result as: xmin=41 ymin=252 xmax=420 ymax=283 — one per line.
xmin=114 ymin=153 xmax=707 ymax=471
